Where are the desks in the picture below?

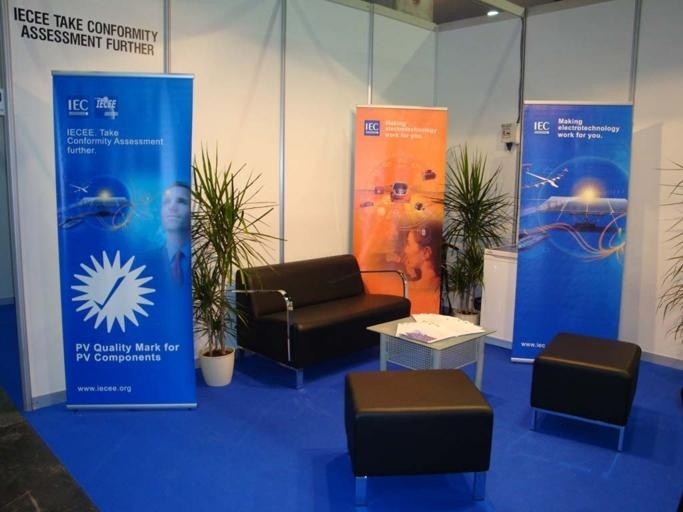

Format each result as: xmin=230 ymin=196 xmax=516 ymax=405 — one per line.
xmin=479 ymin=244 xmax=517 ymax=351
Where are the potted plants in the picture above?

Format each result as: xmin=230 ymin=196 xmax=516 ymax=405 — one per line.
xmin=191 ymin=140 xmax=289 ymax=388
xmin=431 ymin=140 xmax=516 ymax=325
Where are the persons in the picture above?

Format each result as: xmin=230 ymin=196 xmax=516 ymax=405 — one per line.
xmin=401 ymin=221 xmax=441 ymax=285
xmin=141 ymin=182 xmax=190 ymax=292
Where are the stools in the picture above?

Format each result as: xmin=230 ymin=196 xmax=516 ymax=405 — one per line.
xmin=344 ymin=369 xmax=495 ymax=507
xmin=528 ymin=332 xmax=642 ymax=453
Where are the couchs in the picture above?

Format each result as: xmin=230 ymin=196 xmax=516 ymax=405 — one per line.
xmin=223 ymin=254 xmax=412 ymax=390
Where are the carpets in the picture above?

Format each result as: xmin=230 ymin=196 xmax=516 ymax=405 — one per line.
xmin=0 ymin=299 xmax=683 ymax=512
